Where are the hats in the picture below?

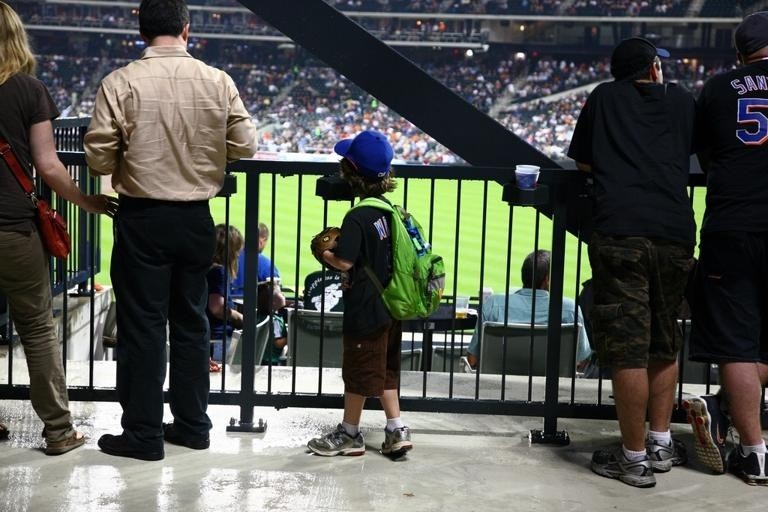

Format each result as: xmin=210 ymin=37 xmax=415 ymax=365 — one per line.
xmin=610 ymin=36 xmax=669 ymax=79
xmin=734 ymin=11 xmax=767 ymax=56
xmin=333 ymin=131 xmax=392 ymax=179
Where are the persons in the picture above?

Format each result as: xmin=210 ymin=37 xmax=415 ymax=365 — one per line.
xmin=205 ymin=222 xmax=243 ymax=368
xmin=686 ymin=10 xmax=768 ymax=487
xmin=567 ymin=33 xmax=699 ymax=489
xmin=300 ymin=269 xmax=347 ymax=312
xmin=15 ymin=3 xmax=742 ymax=170
xmin=468 ymin=250 xmax=594 ymax=375
xmin=256 ymin=276 xmax=290 ymax=366
xmin=306 ymin=129 xmax=414 ymax=458
xmin=229 ymin=224 xmax=287 ymax=300
xmin=82 ymin=1 xmax=260 ymax=461
xmin=1 ymin=4 xmax=119 ymax=456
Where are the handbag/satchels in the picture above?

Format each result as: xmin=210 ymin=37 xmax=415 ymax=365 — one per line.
xmin=34 ymin=199 xmax=71 ymax=259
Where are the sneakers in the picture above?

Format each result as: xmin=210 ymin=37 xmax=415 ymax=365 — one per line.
xmin=590 ymin=448 xmax=655 ymax=488
xmin=682 ymin=395 xmax=729 ymax=475
xmin=45 ymin=431 xmax=84 ymax=455
xmin=307 ymin=423 xmax=364 ymax=457
xmin=644 ymin=440 xmax=687 ymax=473
xmin=724 ymin=445 xmax=767 ymax=486
xmin=381 ymin=427 xmax=411 ymax=455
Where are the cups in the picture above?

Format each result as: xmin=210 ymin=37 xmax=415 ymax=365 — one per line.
xmin=514 ymin=165 xmax=541 ymax=191
xmin=455 ymin=297 xmax=468 ymax=318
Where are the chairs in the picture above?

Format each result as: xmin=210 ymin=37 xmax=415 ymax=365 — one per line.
xmin=231 ymin=316 xmax=271 ymax=368
xmin=460 ymin=317 xmax=586 ymax=379
xmin=397 ymin=340 xmax=473 ymax=371
xmin=283 ymin=305 xmax=345 ymax=370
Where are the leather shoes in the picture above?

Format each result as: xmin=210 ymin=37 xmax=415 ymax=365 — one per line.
xmin=162 ymin=423 xmax=209 ymax=449
xmin=97 ymin=434 xmax=164 ymax=461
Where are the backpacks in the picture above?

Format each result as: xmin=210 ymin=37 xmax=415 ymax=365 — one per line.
xmin=342 ymin=198 xmax=444 ymax=321
